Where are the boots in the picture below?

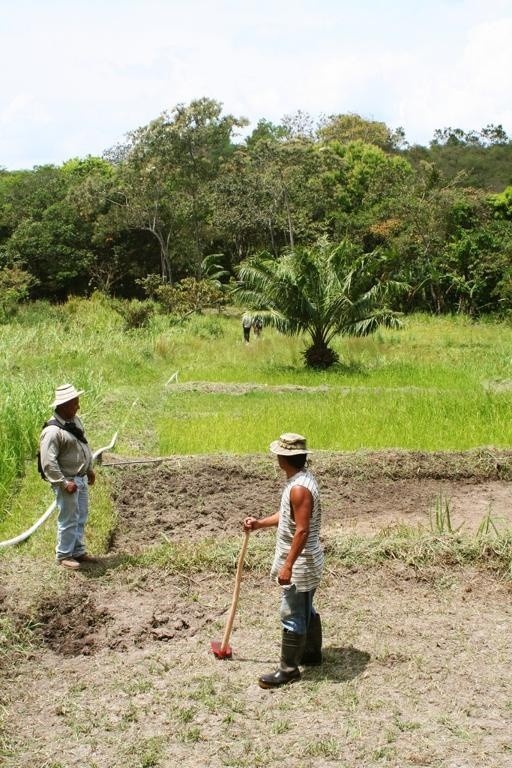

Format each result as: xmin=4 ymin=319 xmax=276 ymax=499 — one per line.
xmin=256 ymin=627 xmax=302 ymax=690
xmin=300 ymin=612 xmax=322 ymax=665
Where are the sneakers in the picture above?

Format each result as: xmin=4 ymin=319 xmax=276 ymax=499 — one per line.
xmin=60 ymin=557 xmax=81 ymax=570
xmin=72 ymin=550 xmax=97 ymax=563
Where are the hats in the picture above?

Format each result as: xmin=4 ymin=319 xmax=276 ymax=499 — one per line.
xmin=267 ymin=431 xmax=315 ymax=458
xmin=48 ymin=383 xmax=86 ymax=409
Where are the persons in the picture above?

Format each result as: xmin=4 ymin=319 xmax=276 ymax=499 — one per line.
xmin=242 ymin=447 xmax=326 ymax=692
xmin=37 ymin=394 xmax=99 ymax=571
xmin=252 ymin=314 xmax=262 ymax=338
xmin=241 ymin=311 xmax=253 ymax=345
